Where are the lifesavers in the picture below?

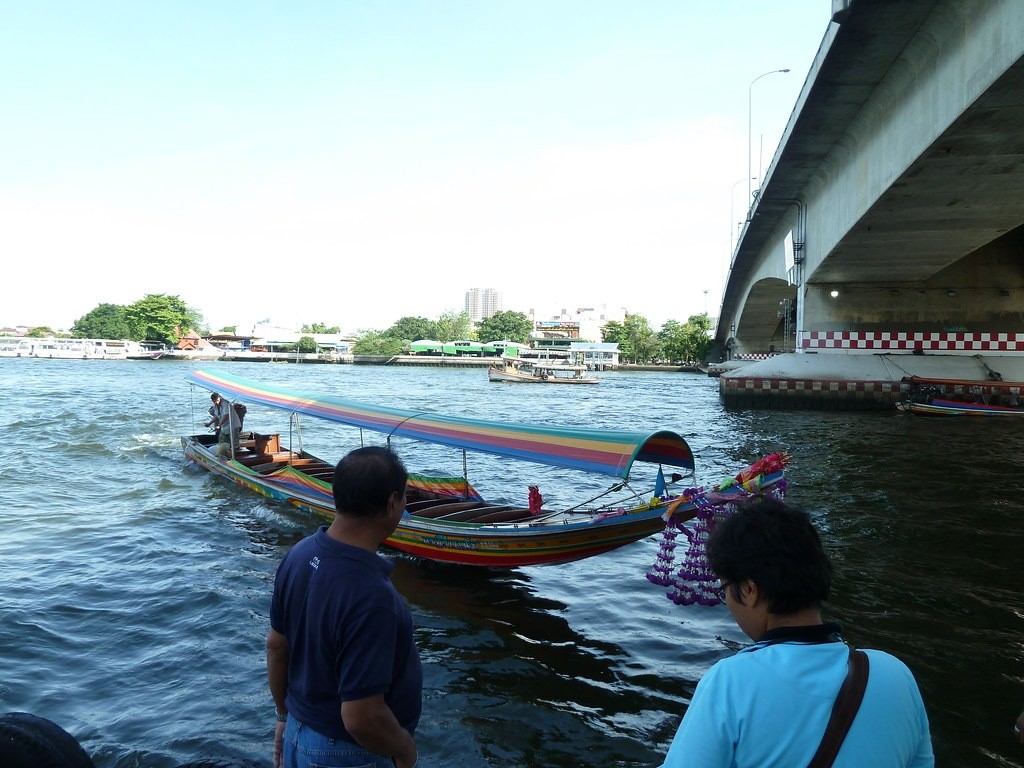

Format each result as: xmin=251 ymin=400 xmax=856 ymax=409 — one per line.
xmin=542 ymin=375 xmax=549 ymax=381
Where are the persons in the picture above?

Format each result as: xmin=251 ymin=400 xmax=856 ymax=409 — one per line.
xmin=906 ymin=384 xmax=1019 ymax=407
xmin=204 ymin=393 xmax=241 ymax=449
xmin=659 ymin=501 xmax=935 ymax=768
xmin=505 ymin=350 xmax=583 ymax=377
xmin=266 ymin=447 xmax=422 ymax=768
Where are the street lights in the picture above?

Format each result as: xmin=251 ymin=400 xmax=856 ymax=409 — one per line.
xmin=729 ymin=176 xmax=757 ymax=264
xmin=745 ymin=68 xmax=792 ymax=208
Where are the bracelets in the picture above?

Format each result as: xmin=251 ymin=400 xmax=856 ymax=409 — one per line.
xmin=413 ymin=751 xmax=419 ymax=768
xmin=274 ymin=706 xmax=288 ymax=722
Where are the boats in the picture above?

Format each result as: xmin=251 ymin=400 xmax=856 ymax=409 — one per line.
xmin=895 ymin=374 xmax=1024 ymax=419
xmin=179 ymin=368 xmax=793 ymax=572
xmin=486 ymin=357 xmax=601 ymax=385
